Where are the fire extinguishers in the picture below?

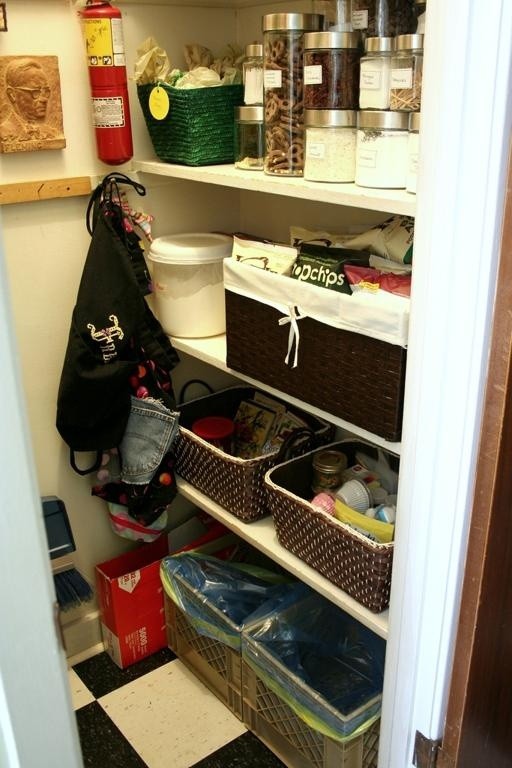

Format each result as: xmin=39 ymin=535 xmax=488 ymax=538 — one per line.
xmin=78 ymin=0 xmax=134 ymax=166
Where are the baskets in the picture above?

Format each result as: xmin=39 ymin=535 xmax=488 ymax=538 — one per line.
xmin=223 ymin=282 xmax=404 ymax=443
xmin=262 ymin=435 xmax=399 ymax=614
xmin=134 ymin=74 xmax=244 ymax=165
xmin=171 ymin=378 xmax=334 ymax=524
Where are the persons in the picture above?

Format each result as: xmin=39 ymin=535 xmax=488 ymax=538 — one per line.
xmin=1 ymin=59 xmax=63 ymax=142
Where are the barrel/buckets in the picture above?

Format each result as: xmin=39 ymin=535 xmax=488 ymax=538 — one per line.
xmin=145 ymin=231 xmax=232 ymax=340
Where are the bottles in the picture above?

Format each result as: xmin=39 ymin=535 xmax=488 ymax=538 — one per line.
xmin=234 ymin=0 xmax=422 ymax=196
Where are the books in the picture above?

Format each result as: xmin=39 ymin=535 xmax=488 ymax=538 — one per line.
xmin=226 ymin=392 xmax=311 ymax=460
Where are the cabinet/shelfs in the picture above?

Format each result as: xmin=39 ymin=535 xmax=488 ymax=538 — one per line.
xmin=122 ymin=0 xmax=429 ymax=768
